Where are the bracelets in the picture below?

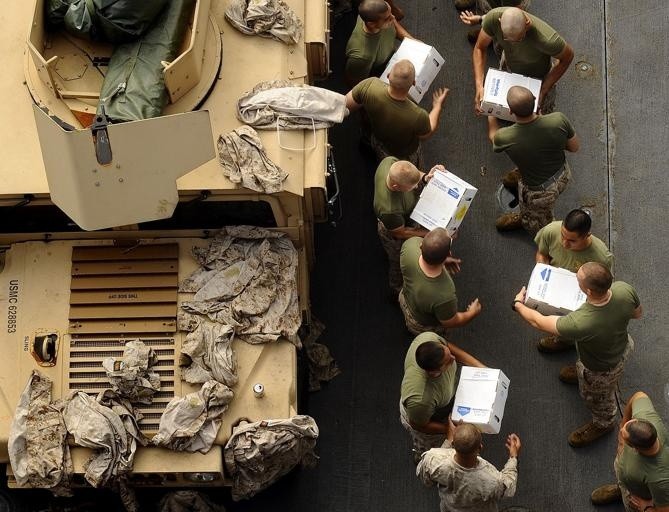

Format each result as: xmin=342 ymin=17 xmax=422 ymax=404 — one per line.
xmin=422 ymin=173 xmax=429 ymax=187
xmin=511 ymin=300 xmax=523 ymax=311
xmin=643 ymin=504 xmax=653 ymax=511
xmin=477 ymin=15 xmax=485 ymax=26
xmin=508 ymin=454 xmax=518 ymax=461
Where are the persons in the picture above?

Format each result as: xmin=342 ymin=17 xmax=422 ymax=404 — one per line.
xmin=534 ymin=207 xmax=614 ymax=355
xmin=508 ymin=260 xmax=644 ymax=452
xmin=415 ymin=411 xmax=524 ymax=511
xmin=399 ymin=329 xmax=493 ymax=470
xmin=486 ymin=85 xmax=582 ymax=238
xmin=344 ymin=59 xmax=450 ymax=173
xmin=472 ymin=5 xmax=576 ymax=127
xmin=345 ymin=0 xmax=424 ymax=92
xmin=371 ymin=154 xmax=459 ymax=297
xmin=589 ymin=389 xmax=669 ymax=512
xmin=336 ymin=1 xmax=407 ymax=37
xmin=396 ymin=226 xmax=484 ymax=342
xmin=457 ymin=0 xmax=537 ymax=47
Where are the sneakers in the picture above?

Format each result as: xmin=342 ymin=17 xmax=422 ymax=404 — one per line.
xmin=495 ymin=211 xmax=525 ymax=231
xmin=560 ymin=365 xmax=579 ymax=385
xmin=567 ymin=418 xmax=617 ymax=448
xmin=454 ymin=0 xmax=476 ymax=12
xmin=536 ymin=334 xmax=575 ymax=354
xmin=591 ymin=483 xmax=621 ymax=506
xmin=384 ymin=0 xmax=405 ymax=23
xmin=468 ymin=29 xmax=494 ymax=48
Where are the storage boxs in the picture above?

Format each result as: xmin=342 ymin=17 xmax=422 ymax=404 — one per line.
xmin=450 ymin=365 xmax=512 ymax=436
xmin=408 ymin=167 xmax=478 ymax=242
xmin=477 ymin=64 xmax=543 ymax=124
xmin=377 ymin=35 xmax=447 ymax=104
xmin=523 ymin=260 xmax=588 ymax=321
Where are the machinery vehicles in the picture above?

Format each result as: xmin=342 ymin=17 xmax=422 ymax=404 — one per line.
xmin=1 ymin=0 xmax=334 ymax=494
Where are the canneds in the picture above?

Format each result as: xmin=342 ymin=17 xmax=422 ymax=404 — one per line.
xmin=253 ymin=383 xmax=264 ymax=398
